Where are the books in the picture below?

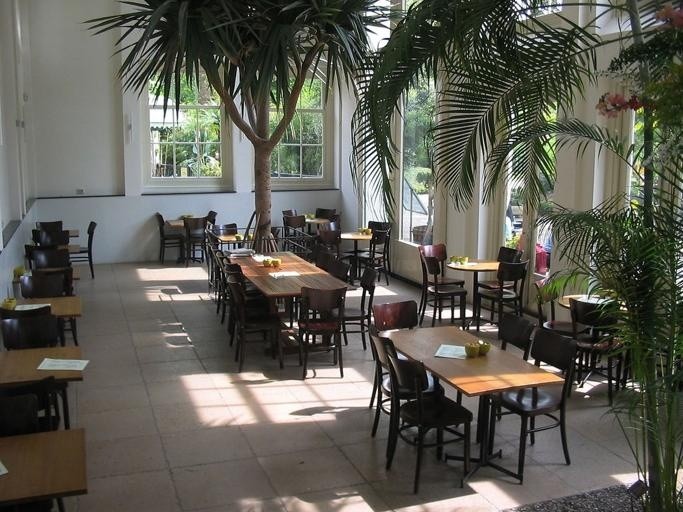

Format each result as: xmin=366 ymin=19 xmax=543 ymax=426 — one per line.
xmin=225 ymin=249 xmax=250 ymax=257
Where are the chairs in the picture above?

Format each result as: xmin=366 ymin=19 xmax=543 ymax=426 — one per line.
xmin=0 ymin=220 xmax=99 ymax=512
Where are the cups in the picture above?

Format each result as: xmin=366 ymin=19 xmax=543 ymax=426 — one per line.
xmin=13 ymin=263 xmax=26 ymax=277
xmin=477 ymin=341 xmax=490 ymax=355
xmin=459 ymin=256 xmax=468 ymax=265
xmin=1 ymin=297 xmax=16 ymax=310
xmin=450 ymin=256 xmax=459 ymax=264
xmin=301 ymin=213 xmax=314 ymax=220
xmin=234 ymin=234 xmax=242 ymax=240
xmin=464 ymin=343 xmax=479 ymax=357
xmin=358 ymin=228 xmax=370 ymax=235
xmin=271 ymin=259 xmax=281 ymax=266
xmin=263 ymin=259 xmax=271 ymax=266
xmin=247 ymin=234 xmax=253 ymax=240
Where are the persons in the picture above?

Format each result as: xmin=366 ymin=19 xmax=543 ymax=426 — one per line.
xmin=544 ymin=230 xmax=553 ymax=269
xmin=505 ymin=216 xmax=514 ymax=242
xmin=506 ymin=203 xmax=514 ymax=222
